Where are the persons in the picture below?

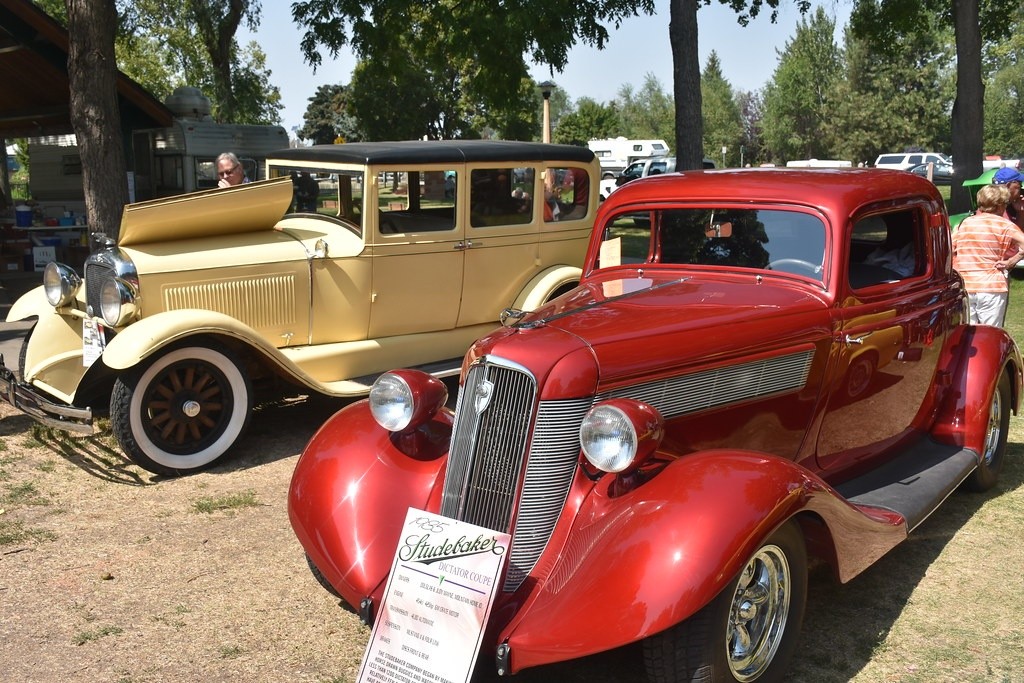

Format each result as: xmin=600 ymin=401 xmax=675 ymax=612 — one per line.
xmin=291 ymin=170 xmax=318 ymax=214
xmin=513 ymin=139 xmax=593 ymax=222
xmin=950 ymin=167 xmax=1024 ymax=329
xmin=212 ymin=153 xmax=250 ymax=191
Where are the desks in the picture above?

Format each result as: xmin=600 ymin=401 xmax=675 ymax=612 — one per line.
xmin=14 ymin=223 xmax=87 ymax=265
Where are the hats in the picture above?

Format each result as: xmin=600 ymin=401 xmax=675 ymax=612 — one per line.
xmin=993 ymin=167 xmax=1024 ymax=187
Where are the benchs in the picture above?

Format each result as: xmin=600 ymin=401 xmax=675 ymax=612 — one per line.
xmin=846 ymin=241 xmax=915 ymax=288
xmin=379 ymin=212 xmax=453 ymax=234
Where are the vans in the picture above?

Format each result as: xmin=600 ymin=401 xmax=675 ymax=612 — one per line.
xmin=874 ymin=153 xmax=954 ymax=171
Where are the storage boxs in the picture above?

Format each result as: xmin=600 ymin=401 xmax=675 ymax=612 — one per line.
xmin=30 ymin=231 xmax=82 ymax=272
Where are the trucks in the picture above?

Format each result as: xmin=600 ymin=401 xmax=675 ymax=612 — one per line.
xmin=588 ymin=136 xmax=671 ymax=180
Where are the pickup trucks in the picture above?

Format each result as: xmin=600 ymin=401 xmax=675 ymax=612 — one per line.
xmin=983 ymin=159 xmax=1021 ymax=173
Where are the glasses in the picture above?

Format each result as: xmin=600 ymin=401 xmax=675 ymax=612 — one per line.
xmin=219 ymin=163 xmax=240 ymax=178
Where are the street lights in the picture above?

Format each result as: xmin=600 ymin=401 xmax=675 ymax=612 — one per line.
xmin=536 ymin=80 xmax=557 ymax=143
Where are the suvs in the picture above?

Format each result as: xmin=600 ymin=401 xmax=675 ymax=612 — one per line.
xmin=599 ymin=158 xmax=715 ymax=200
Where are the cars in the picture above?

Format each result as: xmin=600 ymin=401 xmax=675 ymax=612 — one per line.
xmin=287 ymin=166 xmax=1024 ymax=683
xmin=356 ymin=170 xmax=396 ymax=182
xmin=906 ymin=164 xmax=955 ymax=185
xmin=0 ymin=139 xmax=654 ymax=482
xmin=513 ymin=167 xmax=536 ymax=184
